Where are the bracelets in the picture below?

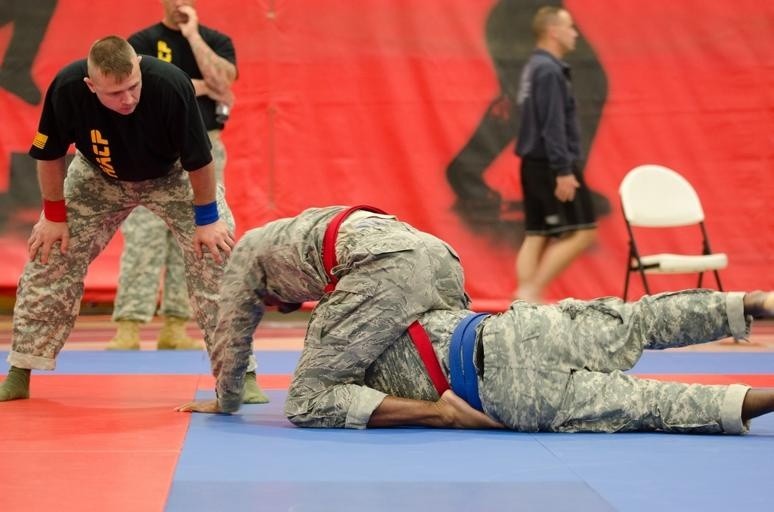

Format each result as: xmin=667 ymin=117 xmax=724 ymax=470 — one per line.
xmin=38 ymin=196 xmax=70 ymax=226
xmin=191 ymin=199 xmax=221 ymax=224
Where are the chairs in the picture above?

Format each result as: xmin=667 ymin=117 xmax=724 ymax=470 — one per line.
xmin=617 ymin=161 xmax=729 ymax=307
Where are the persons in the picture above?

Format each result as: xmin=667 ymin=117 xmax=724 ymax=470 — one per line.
xmin=366 ymin=287 xmax=774 ymax=434
xmin=107 ymin=3 xmax=238 ymax=348
xmin=0 ymin=35 xmax=270 ymax=404
xmin=512 ymin=3 xmax=603 ymax=314
xmin=169 ymin=204 xmax=507 ymax=430
